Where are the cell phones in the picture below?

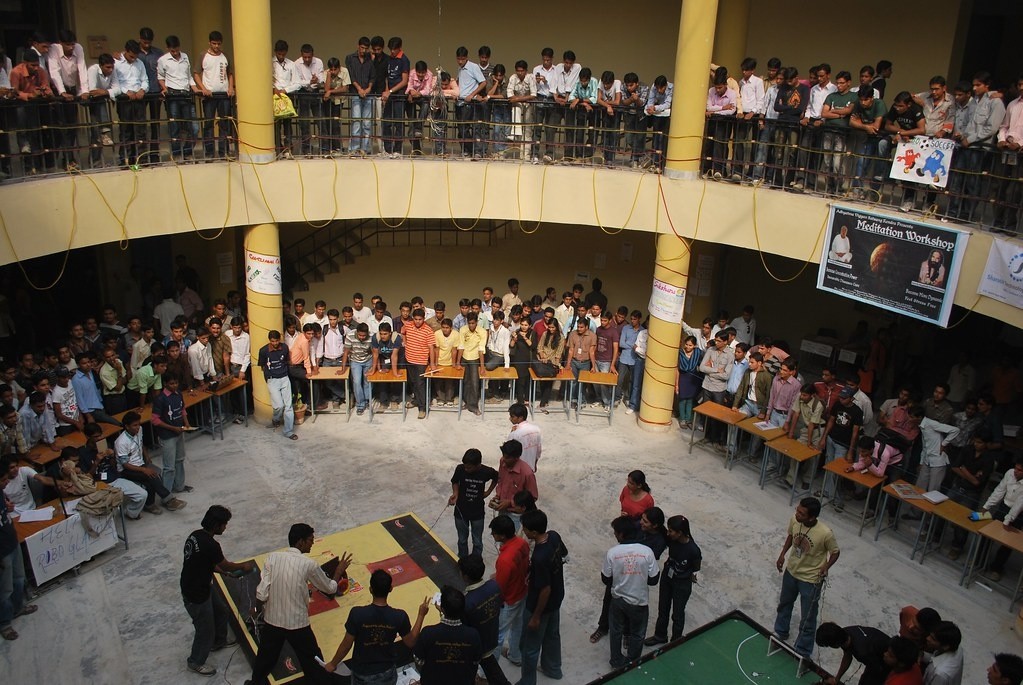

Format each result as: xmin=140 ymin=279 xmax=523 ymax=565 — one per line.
xmin=537 ymin=72 xmax=540 ymax=77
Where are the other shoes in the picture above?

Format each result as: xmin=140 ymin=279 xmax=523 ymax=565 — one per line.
xmin=590 ymin=627 xmax=607 ymax=642
xmin=148 ymin=505 xmax=162 ymax=515
xmin=644 ymin=636 xmax=667 ymax=646
xmin=475 ymin=673 xmax=489 ymax=685
xmin=208 ymin=412 xmax=246 ymax=425
xmin=356 ymin=399 xmax=635 ymax=420
xmin=680 ymin=420 xmax=703 ymax=432
xmin=289 ymin=434 xmax=298 ymax=440
xmin=272 ymin=420 xmax=280 ymax=427
xmin=501 ymin=646 xmax=521 ymax=667
xmin=537 ymin=662 xmax=544 ymax=672
xmin=181 ymin=484 xmax=193 ymax=492
xmin=623 ymin=635 xmax=629 ymax=648
xmin=715 ymin=444 xmax=1002 ymax=584
xmin=167 ymin=498 xmax=187 ymax=510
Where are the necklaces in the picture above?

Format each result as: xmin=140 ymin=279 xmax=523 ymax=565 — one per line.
xmin=539 ymin=533 xmax=548 ymax=545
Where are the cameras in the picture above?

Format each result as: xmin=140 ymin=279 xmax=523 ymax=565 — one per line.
xmin=37 ymin=88 xmax=46 ymax=92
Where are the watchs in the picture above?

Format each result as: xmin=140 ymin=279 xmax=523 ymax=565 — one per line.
xmin=897 ymin=131 xmax=901 ymax=135
xmin=389 ymin=89 xmax=393 ymax=93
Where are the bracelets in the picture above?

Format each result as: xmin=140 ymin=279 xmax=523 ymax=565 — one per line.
xmin=758 ymin=123 xmax=762 ymax=126
xmin=379 ymin=369 xmax=382 ymax=372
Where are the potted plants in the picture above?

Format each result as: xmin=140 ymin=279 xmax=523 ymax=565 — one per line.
xmin=292 ymin=389 xmax=307 ymax=425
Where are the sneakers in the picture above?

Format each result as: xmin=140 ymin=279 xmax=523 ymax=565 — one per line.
xmin=21 ymin=605 xmax=38 ymax=615
xmin=0 ymin=625 xmax=18 ymax=640
xmin=187 ymin=664 xmax=216 ymax=676
xmin=223 ymin=636 xmax=238 ymax=647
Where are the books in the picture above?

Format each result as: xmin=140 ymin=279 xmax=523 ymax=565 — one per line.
xmin=921 ymin=490 xmax=950 ymax=504
xmin=891 ymin=484 xmax=923 ymax=499
xmin=968 ymin=511 xmax=993 ymax=521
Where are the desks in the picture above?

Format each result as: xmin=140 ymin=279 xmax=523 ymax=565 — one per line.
xmin=26 ymin=436 xmax=77 ymax=476
xmin=728 ymin=415 xmax=788 ymax=486
xmin=821 ymin=458 xmax=1023 ymax=613
xmin=211 ymin=511 xmax=515 ymax=685
xmin=688 ymin=401 xmax=747 ymax=469
xmin=577 ymin=370 xmax=618 ymax=426
xmin=527 ymin=368 xmax=576 ymax=422
xmin=477 ymin=366 xmax=518 ymax=420
xmin=799 ymin=333 xmax=840 ymax=368
xmin=760 ymin=434 xmax=821 ymax=506
xmin=367 ymin=368 xmax=407 ymax=422
xmin=11 ymin=480 xmax=129 ymax=576
xmin=307 ymin=367 xmax=352 ymax=422
xmin=181 ymin=388 xmax=216 ymax=441
xmin=584 ymin=609 xmax=848 ymax=685
xmin=112 ymin=404 xmax=157 ymax=456
xmin=424 ymin=365 xmax=465 ymax=420
xmin=197 ymin=375 xmax=249 ymax=441
xmin=64 ymin=415 xmax=124 ymax=447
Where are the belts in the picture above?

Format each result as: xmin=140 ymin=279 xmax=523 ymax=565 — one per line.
xmin=773 ymin=408 xmax=789 ymax=415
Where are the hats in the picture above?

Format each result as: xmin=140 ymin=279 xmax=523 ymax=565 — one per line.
xmin=56 ymin=366 xmax=72 ymax=377
xmin=838 ymin=386 xmax=852 ymax=398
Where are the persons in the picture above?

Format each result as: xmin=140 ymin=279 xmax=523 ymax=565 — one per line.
xmin=259 ymin=331 xmax=299 ymax=440
xmin=589 ymin=507 xmax=669 ymax=646
xmin=179 ymin=505 xmax=255 ymax=677
xmin=0 ymin=253 xmax=1023 ymax=644
xmin=324 ymin=570 xmax=432 ymax=684
xmin=602 ymin=515 xmax=661 ymax=669
xmin=770 ymin=496 xmax=840 ymax=658
xmin=619 ymin=470 xmax=654 ymax=520
xmin=987 ymin=652 xmax=1023 ymax=685
xmin=919 ymin=250 xmax=945 ymax=288
xmin=815 ymin=605 xmax=964 ymax=685
xmin=153 ymin=373 xmax=194 ymax=494
xmin=645 ymin=515 xmax=703 ymax=645
xmin=415 ymin=403 xmax=570 ymax=685
xmin=245 ymin=523 xmax=354 ymax=685
xmin=828 ymin=227 xmax=853 ymax=263
xmin=1 ymin=26 xmax=1023 ymax=237
